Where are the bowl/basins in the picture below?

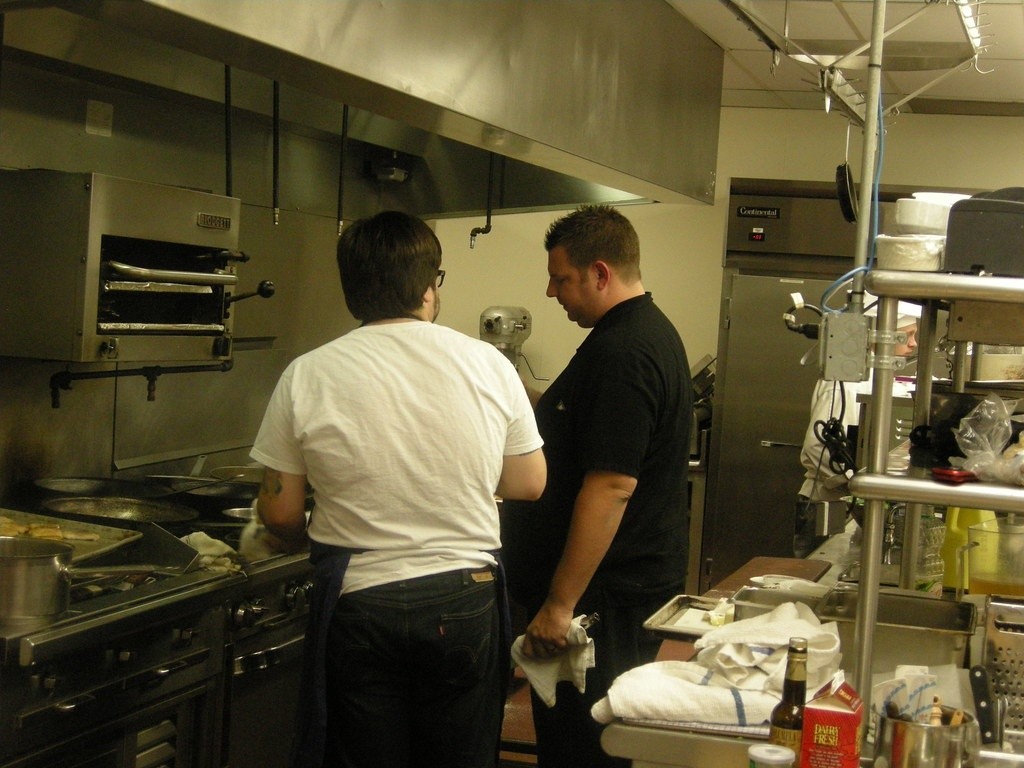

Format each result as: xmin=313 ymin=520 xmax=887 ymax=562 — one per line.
xmin=875 ymin=191 xmax=972 ymax=272
xmin=907 ymin=390 xmax=1023 ymax=425
xmin=980 ymin=353 xmax=1024 ymax=380
xmin=966 ymin=516 xmax=1024 ymax=598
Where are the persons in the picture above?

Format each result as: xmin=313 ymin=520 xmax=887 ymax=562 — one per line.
xmin=249 ymin=210 xmax=547 ymax=768
xmin=792 ymin=290 xmax=921 ymax=560
xmin=495 ymin=206 xmax=694 ymax=768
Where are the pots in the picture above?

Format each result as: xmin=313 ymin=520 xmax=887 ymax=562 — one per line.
xmin=33 ymin=455 xmax=316 ymax=536
xmin=0 ymin=465 xmax=271 ymax=632
xmin=0 ymin=537 xmax=182 ymax=626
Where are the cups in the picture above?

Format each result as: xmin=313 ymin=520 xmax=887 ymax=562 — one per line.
xmin=873 ymin=706 xmax=981 ymax=768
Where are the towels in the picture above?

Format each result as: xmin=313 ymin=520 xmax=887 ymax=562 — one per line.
xmin=240 ymin=498 xmax=312 ymax=563
xmin=590 ymin=601 xmax=839 ymax=726
xmin=178 ymin=531 xmax=238 ymax=571
xmin=510 ymin=614 xmax=596 ymax=709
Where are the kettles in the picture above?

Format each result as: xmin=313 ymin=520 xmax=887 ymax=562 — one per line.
xmin=940 ymin=506 xmax=999 ymax=593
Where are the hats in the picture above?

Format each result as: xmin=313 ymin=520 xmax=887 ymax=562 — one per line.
xmin=847 ymin=285 xmax=922 ymax=332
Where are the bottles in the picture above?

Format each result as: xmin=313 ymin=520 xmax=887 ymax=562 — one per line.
xmin=747 ymin=744 xmax=795 ymax=768
xmin=749 ymin=574 xmax=828 ymax=598
xmin=914 ymin=505 xmax=946 ymax=600
xmin=768 ymin=636 xmax=809 ymax=768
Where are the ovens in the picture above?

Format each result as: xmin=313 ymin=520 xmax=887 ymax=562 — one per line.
xmin=0 ymin=618 xmax=314 ymax=768
xmin=0 ymin=166 xmax=276 ymax=363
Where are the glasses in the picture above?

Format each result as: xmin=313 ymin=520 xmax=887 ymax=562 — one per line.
xmin=436 ymin=269 xmax=445 ymax=287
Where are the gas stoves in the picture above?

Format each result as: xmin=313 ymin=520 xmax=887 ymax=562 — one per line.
xmin=0 ymin=462 xmax=322 ymax=707
